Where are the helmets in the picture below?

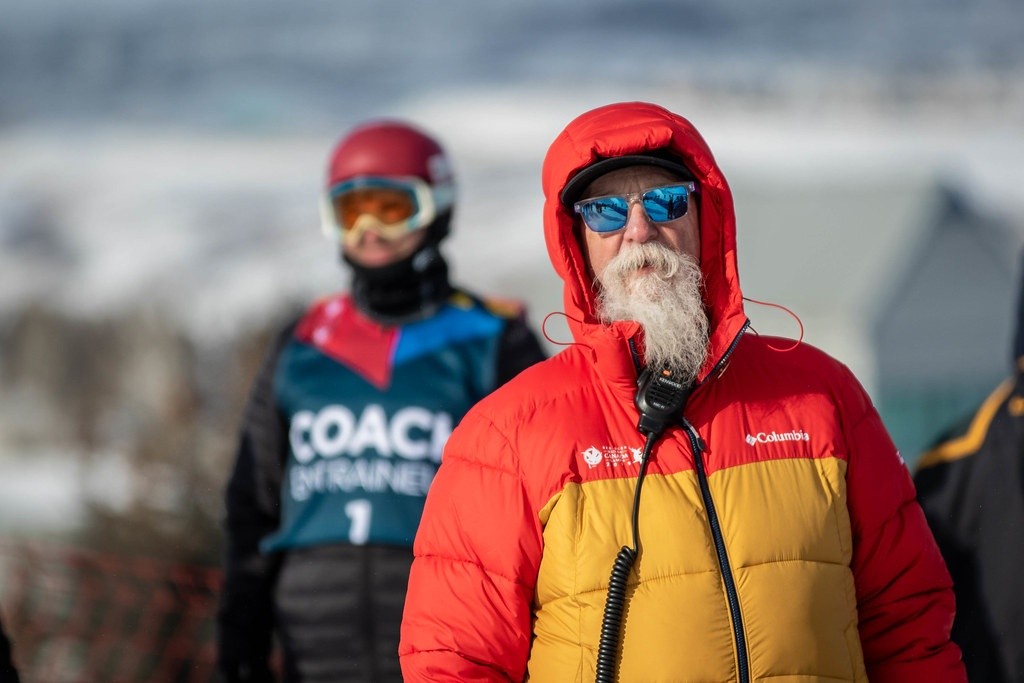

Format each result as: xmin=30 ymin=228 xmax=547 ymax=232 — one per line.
xmin=325 ymin=119 xmax=458 ymax=240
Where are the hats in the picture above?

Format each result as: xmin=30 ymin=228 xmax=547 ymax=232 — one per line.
xmin=557 ymin=141 xmax=700 ymax=211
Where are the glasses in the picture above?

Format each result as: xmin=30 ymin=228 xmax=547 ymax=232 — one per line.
xmin=317 ymin=171 xmax=458 ymax=245
xmin=567 ymin=179 xmax=699 ymax=234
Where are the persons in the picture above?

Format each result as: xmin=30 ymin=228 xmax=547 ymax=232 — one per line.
xmin=214 ymin=119 xmax=548 ymax=683
xmin=396 ymin=102 xmax=969 ymax=683
